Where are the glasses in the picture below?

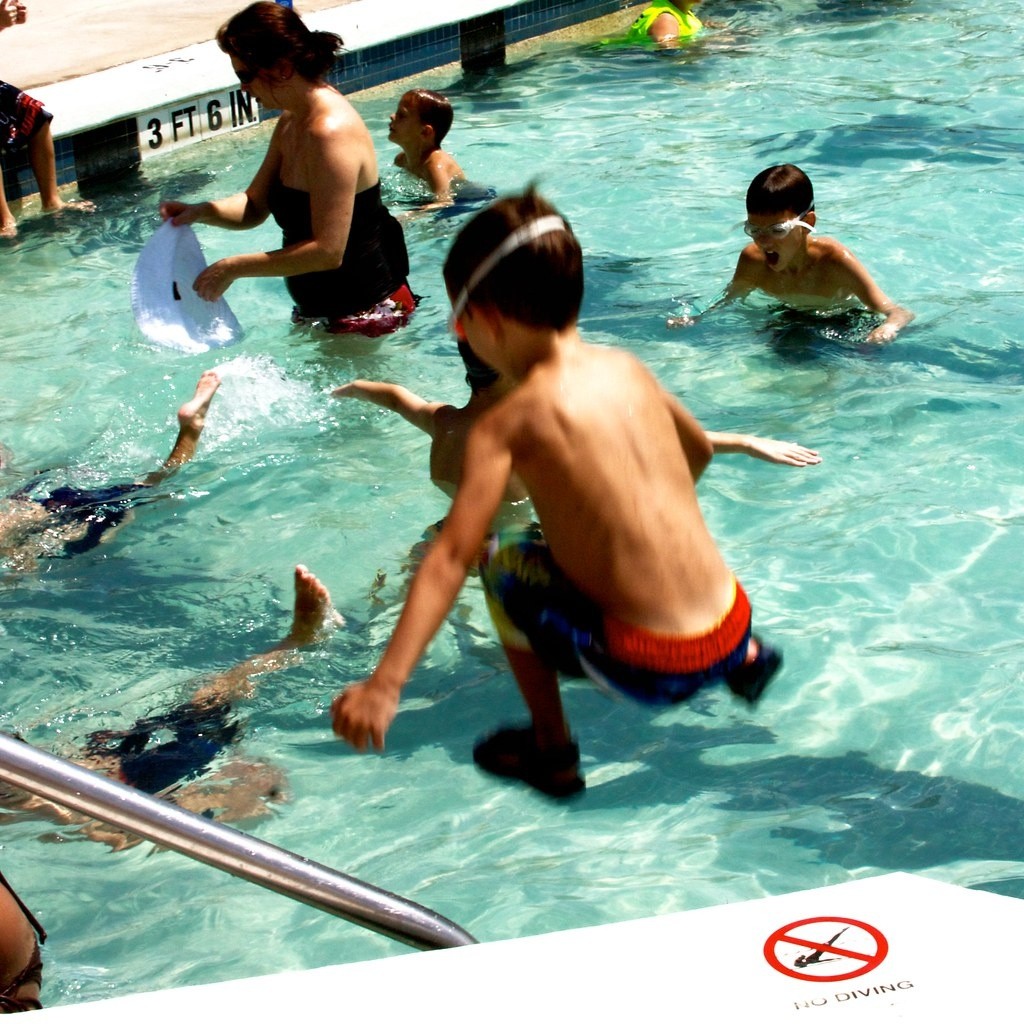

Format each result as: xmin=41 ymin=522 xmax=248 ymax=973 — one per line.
xmin=743 ymin=201 xmax=815 ymax=242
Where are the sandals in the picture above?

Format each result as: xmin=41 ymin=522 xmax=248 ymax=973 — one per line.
xmin=723 ymin=632 xmax=782 ymax=705
xmin=472 ymin=726 xmax=589 ymax=806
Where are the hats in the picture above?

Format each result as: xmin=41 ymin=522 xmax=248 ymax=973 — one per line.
xmin=133 ymin=220 xmax=247 ymax=357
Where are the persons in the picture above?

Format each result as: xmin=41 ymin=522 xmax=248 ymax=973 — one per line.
xmin=327 ymin=336 xmax=824 ymax=566
xmin=0 ymin=875 xmax=42 ymax=1014
xmin=0 ymin=0 xmax=98 ymax=240
xmin=262 ymin=564 xmax=347 ymax=662
xmin=327 ymin=174 xmax=784 ymax=802
xmin=165 ymin=368 xmax=223 ymax=465
xmin=630 ymin=0 xmax=706 ymax=49
xmin=667 ymin=165 xmax=916 ymax=348
xmin=157 ymin=2 xmax=421 ymax=338
xmin=386 ymin=90 xmax=491 ymax=235
